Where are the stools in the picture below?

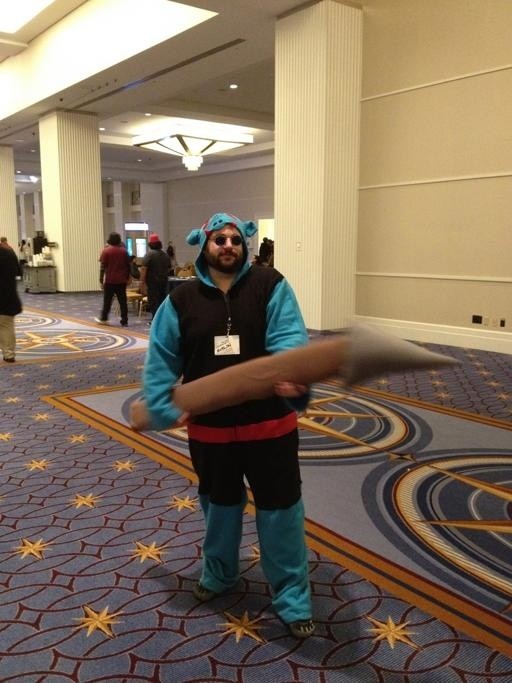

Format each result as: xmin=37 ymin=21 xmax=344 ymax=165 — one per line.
xmin=112 ymin=286 xmax=152 ymax=320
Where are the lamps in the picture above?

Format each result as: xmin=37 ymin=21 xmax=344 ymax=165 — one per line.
xmin=129 ymin=121 xmax=258 ymax=173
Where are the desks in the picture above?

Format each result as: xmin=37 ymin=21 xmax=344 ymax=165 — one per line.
xmin=166 ymin=274 xmax=193 ymax=291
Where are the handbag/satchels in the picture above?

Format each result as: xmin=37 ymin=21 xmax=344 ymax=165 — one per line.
xmin=129 ymin=262 xmax=140 ymax=278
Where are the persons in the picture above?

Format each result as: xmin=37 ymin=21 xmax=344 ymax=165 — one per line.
xmin=167 ymin=245 xmax=176 ymax=276
xmin=142 ymin=213 xmax=315 ymax=638
xmin=130 ymin=255 xmax=140 ymax=279
xmin=0 ymin=236 xmax=22 ymax=362
xmin=95 ymin=232 xmax=132 ymax=327
xmin=142 ymin=233 xmax=171 ymax=326
xmin=19 ymin=240 xmax=29 ymax=275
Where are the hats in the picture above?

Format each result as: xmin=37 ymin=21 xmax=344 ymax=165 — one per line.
xmin=149 ymin=235 xmax=159 ymax=242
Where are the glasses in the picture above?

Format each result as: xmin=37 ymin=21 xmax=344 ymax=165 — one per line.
xmin=215 ymin=235 xmax=242 ymax=245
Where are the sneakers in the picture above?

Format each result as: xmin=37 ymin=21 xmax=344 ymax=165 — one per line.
xmin=96 ymin=314 xmax=106 ymax=323
xmin=3 ymin=356 xmax=15 ymax=362
xmin=290 ymin=621 xmax=315 ymax=638
xmin=193 ymin=584 xmax=215 ymax=601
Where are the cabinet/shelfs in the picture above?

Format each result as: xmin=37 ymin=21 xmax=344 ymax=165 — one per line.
xmin=21 ymin=263 xmax=60 ymax=294
